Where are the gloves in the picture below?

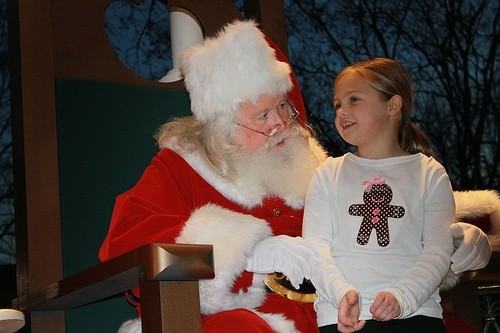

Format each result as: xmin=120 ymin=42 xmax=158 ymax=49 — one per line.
xmin=449 ymin=222 xmax=492 ymax=275
xmin=245 ymin=234 xmax=316 ymax=290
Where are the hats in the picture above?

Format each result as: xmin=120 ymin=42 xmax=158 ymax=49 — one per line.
xmin=180 ymin=19 xmax=307 ymax=130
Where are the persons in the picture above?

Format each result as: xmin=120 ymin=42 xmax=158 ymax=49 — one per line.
xmin=98 ymin=18 xmax=493 ymax=333
xmin=302 ymin=57 xmax=456 ymax=333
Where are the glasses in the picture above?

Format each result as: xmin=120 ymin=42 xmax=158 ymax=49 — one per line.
xmin=233 ymin=100 xmax=300 ymax=137
